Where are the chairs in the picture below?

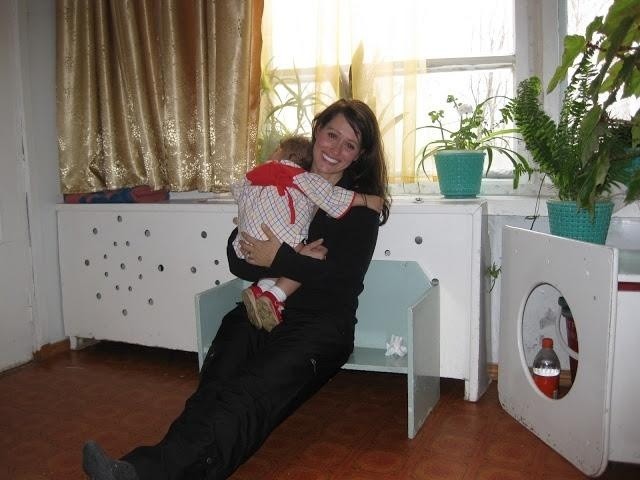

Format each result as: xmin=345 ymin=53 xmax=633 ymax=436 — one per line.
xmin=194 ymin=261 xmax=441 ymax=440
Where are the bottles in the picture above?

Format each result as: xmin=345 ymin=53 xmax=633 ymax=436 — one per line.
xmin=532 ymin=338 xmax=560 ymax=400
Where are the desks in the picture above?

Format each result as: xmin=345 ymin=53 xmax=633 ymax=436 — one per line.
xmin=605 ymin=245 xmax=640 ymax=467
xmin=57 ymin=198 xmax=491 ymax=402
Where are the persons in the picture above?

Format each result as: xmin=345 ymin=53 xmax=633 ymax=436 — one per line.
xmin=83 ymin=98 xmax=393 ymax=479
xmin=230 ymin=136 xmax=385 ymax=332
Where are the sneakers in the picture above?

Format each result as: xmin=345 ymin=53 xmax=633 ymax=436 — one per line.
xmin=82 ymin=440 xmax=136 ymax=479
xmin=242 ymin=285 xmax=264 ymax=330
xmin=256 ymin=291 xmax=286 ymax=333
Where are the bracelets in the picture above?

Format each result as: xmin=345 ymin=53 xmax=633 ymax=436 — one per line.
xmin=361 ymin=193 xmax=368 ymax=207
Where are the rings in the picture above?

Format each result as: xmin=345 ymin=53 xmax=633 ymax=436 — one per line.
xmin=246 ymin=252 xmax=249 ymax=257
